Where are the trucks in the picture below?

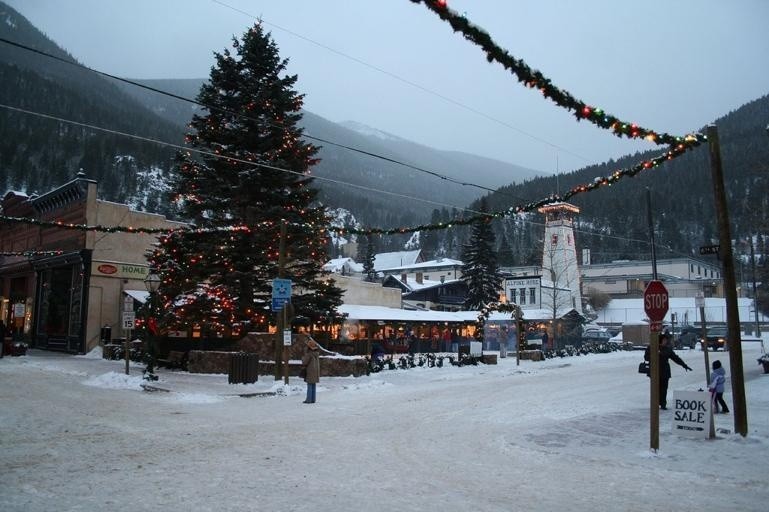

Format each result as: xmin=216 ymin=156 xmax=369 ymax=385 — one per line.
xmin=623 ymin=324 xmax=676 ymax=351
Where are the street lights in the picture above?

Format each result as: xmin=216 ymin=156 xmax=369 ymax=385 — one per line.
xmin=144 ymin=264 xmax=162 ymax=381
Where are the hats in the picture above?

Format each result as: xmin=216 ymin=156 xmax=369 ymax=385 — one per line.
xmin=306 ymin=340 xmax=318 ymax=350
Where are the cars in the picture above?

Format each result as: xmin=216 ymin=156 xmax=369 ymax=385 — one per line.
xmin=608 ymin=332 xmax=623 ymax=349
xmin=701 ymin=327 xmax=729 ymax=351
xmin=662 ymin=325 xmax=697 ymax=349
xmin=581 ymin=331 xmax=613 ymax=348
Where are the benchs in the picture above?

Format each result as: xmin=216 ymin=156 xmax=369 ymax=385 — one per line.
xmin=157 ymin=351 xmax=186 ymax=372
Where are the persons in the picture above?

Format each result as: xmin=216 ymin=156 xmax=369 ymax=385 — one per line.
xmin=301 ymin=340 xmax=320 ymax=403
xmin=709 ymin=360 xmax=729 ymax=414
xmin=644 ymin=335 xmax=692 ymax=410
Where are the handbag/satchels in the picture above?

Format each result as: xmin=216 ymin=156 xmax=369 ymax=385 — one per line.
xmin=299 ymin=367 xmax=306 ymax=378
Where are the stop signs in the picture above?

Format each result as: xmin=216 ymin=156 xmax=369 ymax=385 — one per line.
xmin=645 ymin=280 xmax=669 ymax=321
